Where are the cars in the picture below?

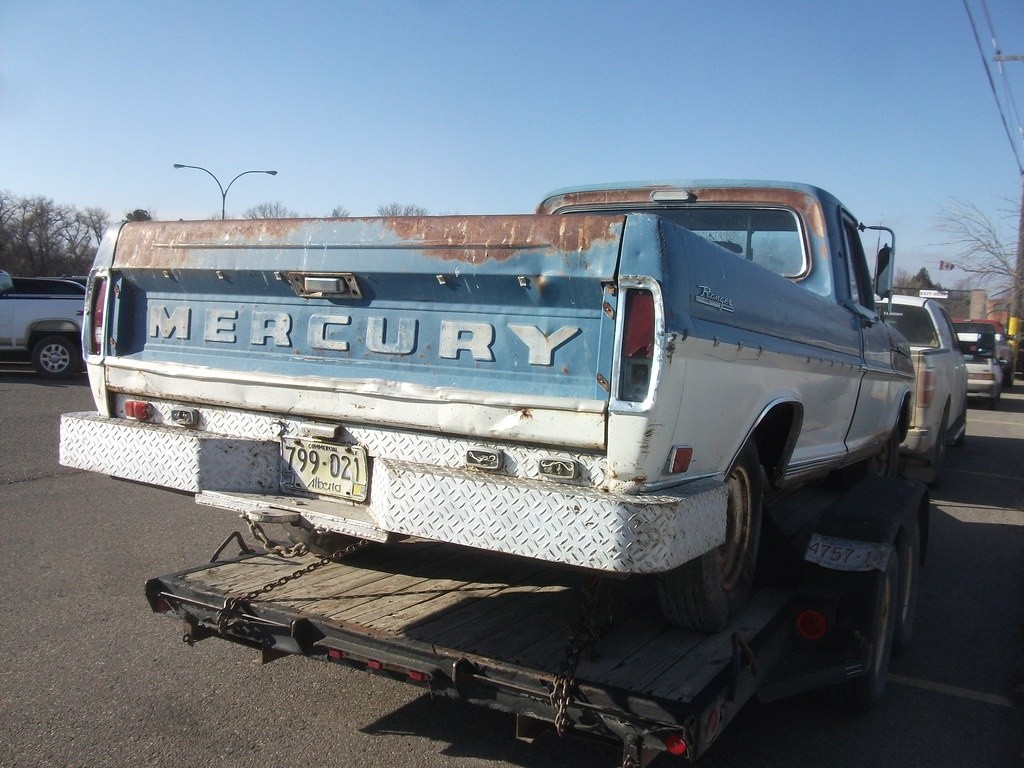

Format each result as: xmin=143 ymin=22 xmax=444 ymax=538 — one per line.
xmin=957 ymin=334 xmax=1007 ymax=409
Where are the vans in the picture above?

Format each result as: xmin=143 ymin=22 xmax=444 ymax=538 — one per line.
xmin=952 ymin=320 xmax=1017 ymax=389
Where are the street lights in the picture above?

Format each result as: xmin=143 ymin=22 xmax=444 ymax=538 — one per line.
xmin=173 ymin=164 xmax=278 ymax=220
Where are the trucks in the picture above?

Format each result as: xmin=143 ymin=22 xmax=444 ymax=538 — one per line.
xmin=143 ymin=458 xmax=938 ymax=768
xmin=0 ymin=270 xmax=91 ymax=378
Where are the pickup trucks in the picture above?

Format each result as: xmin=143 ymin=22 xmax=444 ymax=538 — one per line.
xmin=58 ymin=179 xmax=914 ymax=639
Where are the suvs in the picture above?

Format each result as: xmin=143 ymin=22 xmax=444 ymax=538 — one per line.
xmin=866 ymin=292 xmax=969 ymax=460
